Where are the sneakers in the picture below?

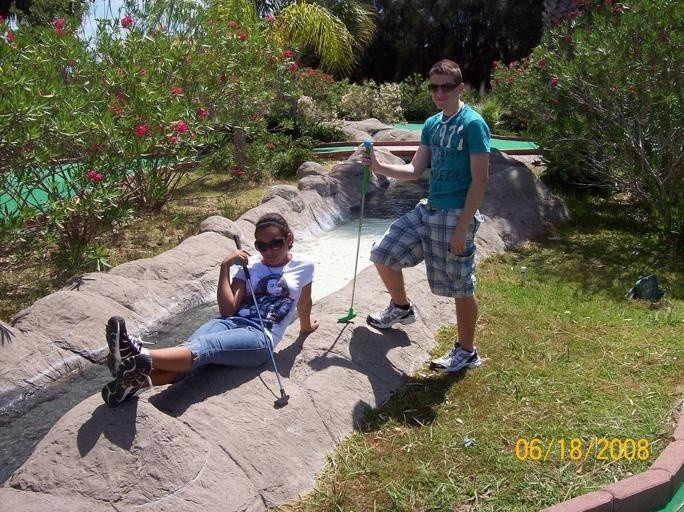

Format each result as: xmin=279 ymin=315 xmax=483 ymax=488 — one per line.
xmin=428 ymin=341 xmax=482 ymax=374
xmin=105 ymin=316 xmax=143 ymax=380
xmin=100 ymin=354 xmax=154 ymax=407
xmin=367 ymin=299 xmax=416 ymax=329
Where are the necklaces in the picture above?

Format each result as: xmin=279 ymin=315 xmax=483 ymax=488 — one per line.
xmin=267 ymin=263 xmax=286 ymax=284
xmin=439 ymin=102 xmax=463 ymax=125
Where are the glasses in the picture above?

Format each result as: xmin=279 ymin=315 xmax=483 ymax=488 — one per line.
xmin=426 ymin=80 xmax=461 ymax=93
xmin=255 ymin=237 xmax=285 ymax=253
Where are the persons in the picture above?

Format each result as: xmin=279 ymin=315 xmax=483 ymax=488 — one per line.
xmin=236 ymin=272 xmax=292 ymax=332
xmin=358 ymin=59 xmax=490 ymax=371
xmin=101 ymin=213 xmax=317 ymax=408
